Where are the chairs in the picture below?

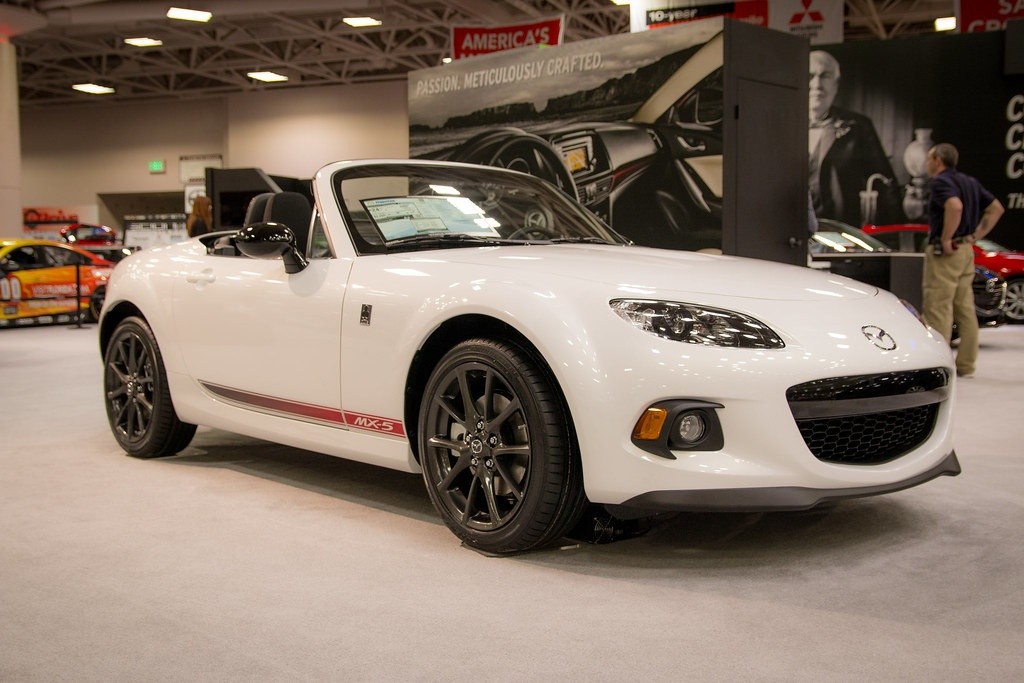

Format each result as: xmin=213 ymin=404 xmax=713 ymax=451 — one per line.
xmin=262 ymin=192 xmax=310 ymax=258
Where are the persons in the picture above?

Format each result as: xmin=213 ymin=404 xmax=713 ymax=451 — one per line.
xmin=807 ymin=49 xmax=906 ymax=252
xmin=921 ymin=143 xmax=1004 ymax=378
xmin=186 ymin=196 xmax=221 ymax=254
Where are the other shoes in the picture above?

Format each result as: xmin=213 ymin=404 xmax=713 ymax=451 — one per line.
xmin=957 ymin=370 xmax=974 ymax=378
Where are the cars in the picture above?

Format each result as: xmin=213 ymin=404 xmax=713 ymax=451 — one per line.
xmin=0 ymin=239 xmax=117 ymax=321
xmin=61 ymin=223 xmax=142 ymax=265
xmin=970 ymin=236 xmax=1024 ymax=323
xmin=808 ymin=219 xmax=1008 ymax=342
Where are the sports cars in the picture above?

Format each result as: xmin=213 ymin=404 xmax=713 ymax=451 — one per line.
xmin=99 ymin=159 xmax=964 ymax=558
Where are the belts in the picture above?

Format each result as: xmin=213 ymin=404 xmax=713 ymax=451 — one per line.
xmin=928 ymin=239 xmax=965 ymax=246
